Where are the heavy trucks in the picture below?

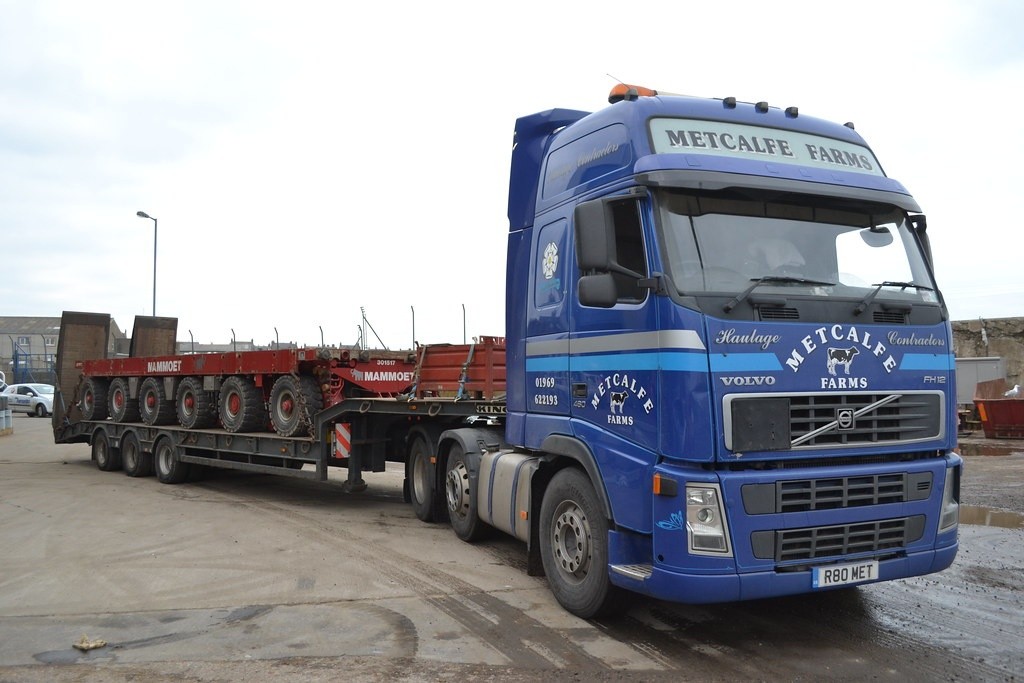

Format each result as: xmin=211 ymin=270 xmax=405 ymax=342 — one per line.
xmin=50 ymin=76 xmax=967 ymax=617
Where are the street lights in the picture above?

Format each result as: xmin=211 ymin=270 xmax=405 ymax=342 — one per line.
xmin=137 ymin=211 xmax=157 ymax=315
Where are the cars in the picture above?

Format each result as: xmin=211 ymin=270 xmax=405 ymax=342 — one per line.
xmin=0 ymin=382 xmax=55 ymax=417
